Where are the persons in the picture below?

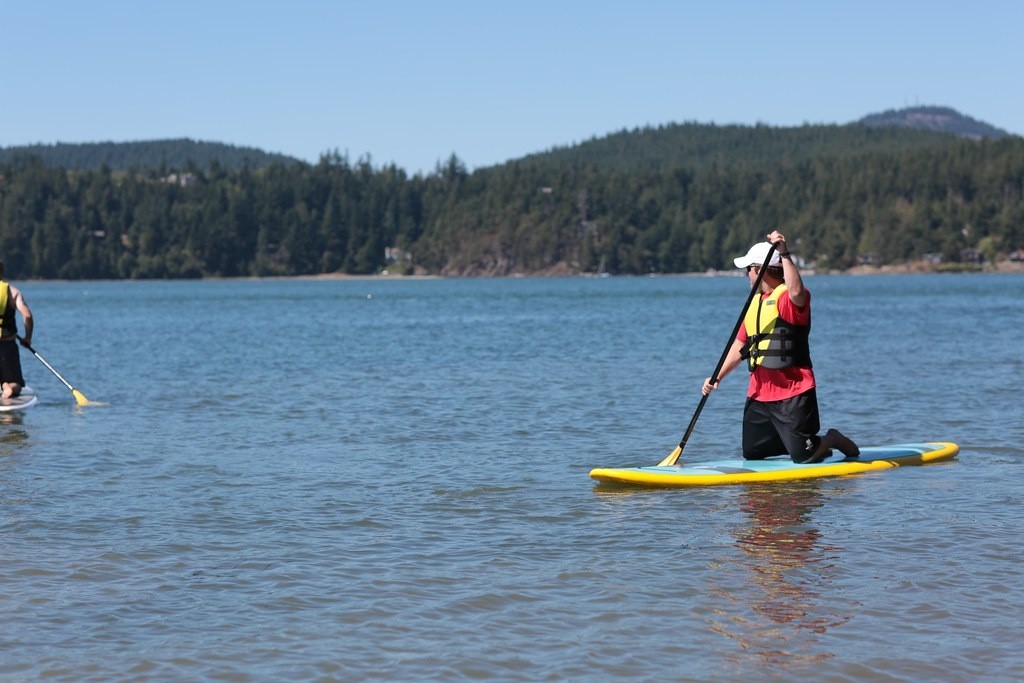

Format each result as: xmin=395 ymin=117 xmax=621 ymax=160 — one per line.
xmin=0 ymin=264 xmax=34 ymax=400
xmin=702 ymin=231 xmax=860 ymax=464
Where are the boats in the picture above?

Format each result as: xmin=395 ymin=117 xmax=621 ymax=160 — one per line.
xmin=588 ymin=441 xmax=961 ymax=488
xmin=1 ymin=384 xmax=39 ymax=411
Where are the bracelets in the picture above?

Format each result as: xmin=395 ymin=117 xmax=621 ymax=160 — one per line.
xmin=779 ymin=252 xmax=794 ymax=259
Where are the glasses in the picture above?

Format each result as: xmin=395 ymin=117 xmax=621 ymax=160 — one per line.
xmin=746 ymin=265 xmax=758 ymax=271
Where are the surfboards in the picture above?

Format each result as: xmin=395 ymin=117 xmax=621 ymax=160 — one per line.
xmin=589 ymin=440 xmax=959 ymax=487
xmin=0 ymin=383 xmax=37 ymax=411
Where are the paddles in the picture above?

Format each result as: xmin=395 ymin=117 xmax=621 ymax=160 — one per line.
xmin=654 ymin=240 xmax=779 ymax=467
xmin=16 ymin=334 xmax=89 ymax=406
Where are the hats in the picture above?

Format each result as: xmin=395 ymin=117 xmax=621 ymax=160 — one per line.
xmin=733 ymin=242 xmax=783 ymax=270
